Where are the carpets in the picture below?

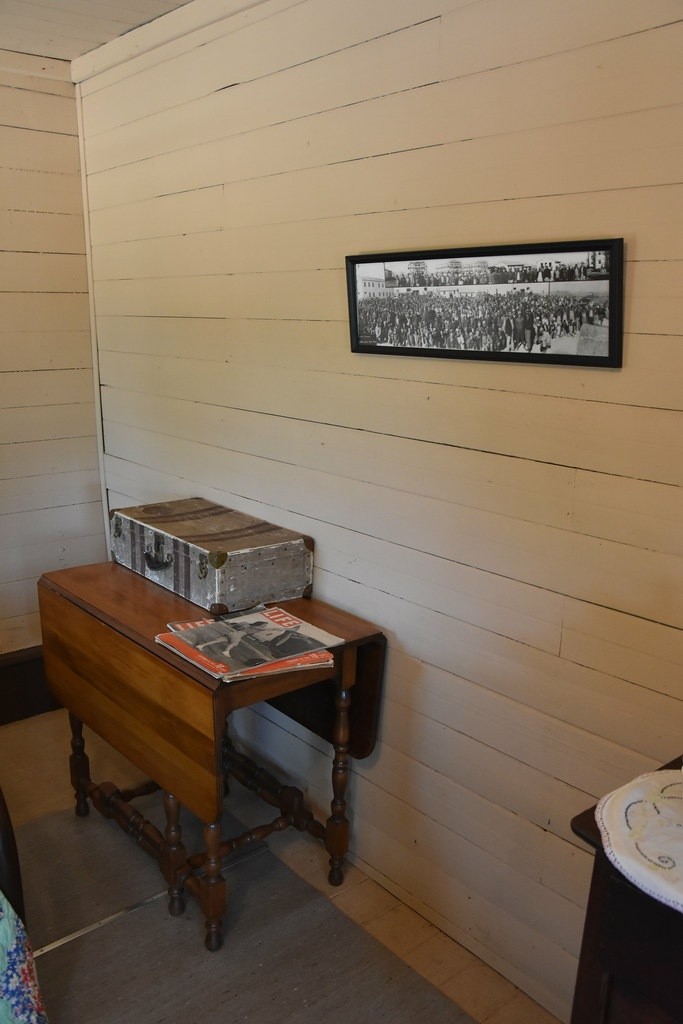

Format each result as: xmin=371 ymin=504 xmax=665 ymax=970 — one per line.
xmin=34 ymin=851 xmax=480 ymax=1024
xmin=13 ymin=782 xmax=269 ymax=952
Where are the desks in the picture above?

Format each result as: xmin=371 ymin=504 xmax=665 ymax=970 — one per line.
xmin=37 ymin=562 xmax=388 ymax=952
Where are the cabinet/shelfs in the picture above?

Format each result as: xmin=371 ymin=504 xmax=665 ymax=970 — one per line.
xmin=569 ymin=755 xmax=683 ymax=1024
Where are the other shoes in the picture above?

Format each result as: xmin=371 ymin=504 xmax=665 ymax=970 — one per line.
xmin=195 ymin=645 xmax=203 ymax=651
xmin=222 ymin=650 xmax=231 ymax=657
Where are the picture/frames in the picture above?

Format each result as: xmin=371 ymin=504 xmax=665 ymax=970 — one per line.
xmin=345 ymin=237 xmax=625 ymax=370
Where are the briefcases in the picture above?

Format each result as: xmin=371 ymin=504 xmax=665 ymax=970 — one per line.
xmin=109 ymin=497 xmax=316 ymax=616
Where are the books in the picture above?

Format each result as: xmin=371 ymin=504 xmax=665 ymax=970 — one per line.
xmin=154 ymin=607 xmax=345 ymax=680
xmin=166 ymin=603 xmax=335 ymax=687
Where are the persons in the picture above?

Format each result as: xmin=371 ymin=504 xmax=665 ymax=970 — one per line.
xmin=356 ymin=258 xmax=607 ymax=354
